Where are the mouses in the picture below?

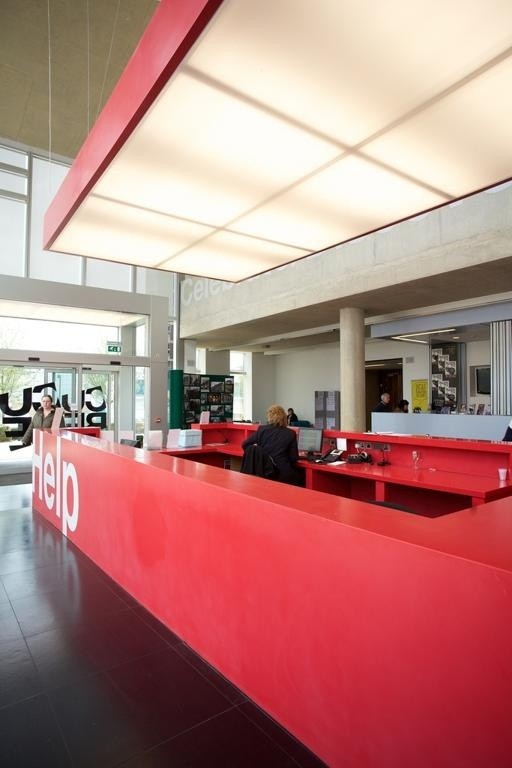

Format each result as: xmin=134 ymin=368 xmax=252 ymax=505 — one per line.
xmin=315 ymin=459 xmax=323 ymax=463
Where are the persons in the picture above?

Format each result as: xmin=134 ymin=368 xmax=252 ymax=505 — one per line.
xmin=286 ymin=408 xmax=298 ymax=426
xmin=22 ymin=395 xmax=65 ymax=445
xmin=376 ymin=392 xmax=394 ymax=413
xmin=242 ymin=404 xmax=299 ymax=486
xmin=395 ymin=399 xmax=410 ymax=413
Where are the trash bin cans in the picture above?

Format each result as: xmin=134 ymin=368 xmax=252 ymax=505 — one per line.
xmin=320 ymin=449 xmax=344 ymax=462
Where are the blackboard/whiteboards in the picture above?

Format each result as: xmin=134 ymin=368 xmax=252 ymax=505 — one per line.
xmin=182 ymin=373 xmax=233 ymax=429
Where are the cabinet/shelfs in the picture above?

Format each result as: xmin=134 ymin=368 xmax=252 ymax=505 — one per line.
xmin=169 ymin=369 xmax=234 ymax=430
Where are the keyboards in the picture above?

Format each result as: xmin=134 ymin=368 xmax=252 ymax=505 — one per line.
xmin=298 ymin=456 xmax=320 ymax=460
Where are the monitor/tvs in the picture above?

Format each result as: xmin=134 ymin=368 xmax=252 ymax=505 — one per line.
xmin=298 ymin=427 xmax=323 ymax=456
xmin=476 ymin=368 xmax=490 ymax=394
xmin=120 ymin=439 xmax=141 ymax=448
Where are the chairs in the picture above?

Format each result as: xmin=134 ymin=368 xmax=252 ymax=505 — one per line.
xmin=242 ymin=445 xmax=280 ymax=482
